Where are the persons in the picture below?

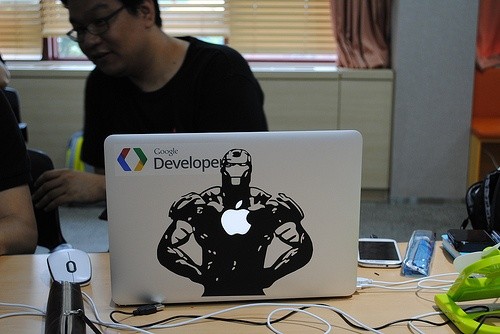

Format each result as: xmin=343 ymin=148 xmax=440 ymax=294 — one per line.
xmin=33 ymin=0 xmax=269 ymax=223
xmin=0 ymin=60 xmax=39 ymax=257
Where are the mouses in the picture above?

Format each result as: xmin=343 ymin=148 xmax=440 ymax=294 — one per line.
xmin=48 ymin=249 xmax=93 ymax=286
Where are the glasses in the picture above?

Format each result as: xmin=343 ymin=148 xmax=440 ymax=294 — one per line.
xmin=66 ymin=6 xmax=124 ymax=43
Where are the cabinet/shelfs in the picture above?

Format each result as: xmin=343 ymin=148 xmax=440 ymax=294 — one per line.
xmin=1 ymin=56 xmax=398 ymax=204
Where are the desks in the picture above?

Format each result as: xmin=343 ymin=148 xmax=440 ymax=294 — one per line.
xmin=0 ymin=240 xmax=500 ymax=334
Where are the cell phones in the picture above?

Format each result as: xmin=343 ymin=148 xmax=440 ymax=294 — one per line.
xmin=358 ymin=237 xmax=403 ymax=267
xmin=448 ymin=229 xmax=496 ymax=252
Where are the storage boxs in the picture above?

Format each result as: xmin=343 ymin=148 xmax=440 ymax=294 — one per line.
xmin=467 ymin=138 xmax=500 ymax=194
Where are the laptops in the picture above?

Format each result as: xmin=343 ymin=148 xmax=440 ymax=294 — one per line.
xmin=104 ymin=129 xmax=362 ymax=306
